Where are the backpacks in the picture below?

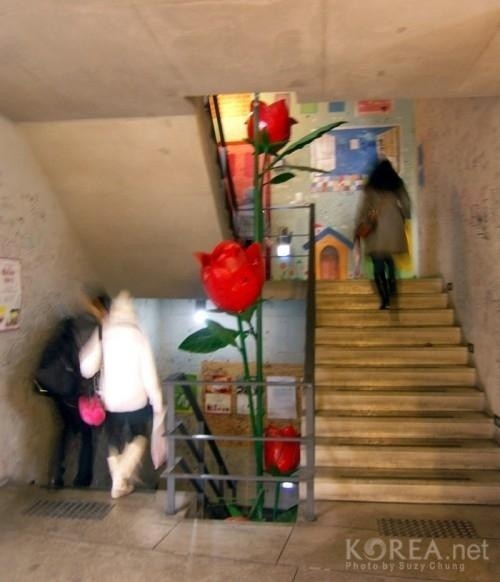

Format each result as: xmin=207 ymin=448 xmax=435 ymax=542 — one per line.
xmin=33 ymin=317 xmax=83 ymax=398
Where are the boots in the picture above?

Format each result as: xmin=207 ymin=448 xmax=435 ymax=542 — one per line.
xmin=376 ymin=277 xmax=390 ymax=310
xmin=107 ymin=455 xmax=135 ymax=498
xmin=389 ymin=272 xmax=395 ymax=294
xmin=118 ymin=435 xmax=149 ymax=486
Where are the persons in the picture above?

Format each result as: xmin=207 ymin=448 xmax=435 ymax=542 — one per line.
xmin=355 ymin=159 xmax=411 ymax=310
xmin=78 ymin=288 xmax=163 ymax=498
xmin=48 ymin=292 xmax=111 ymax=489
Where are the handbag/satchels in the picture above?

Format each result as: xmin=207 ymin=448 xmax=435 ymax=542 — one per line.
xmin=78 ymin=324 xmax=106 ymax=427
xmin=150 ymin=406 xmax=168 ymax=470
xmin=358 ymin=189 xmax=388 ymax=238
